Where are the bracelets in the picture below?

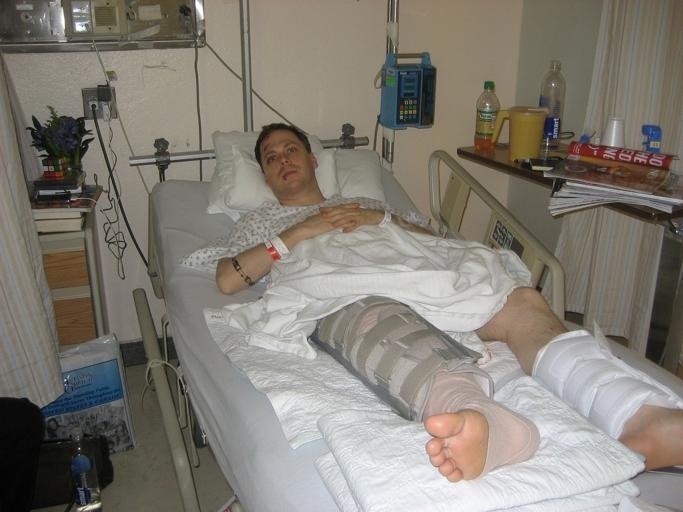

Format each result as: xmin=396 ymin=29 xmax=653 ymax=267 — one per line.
xmin=376 ymin=208 xmax=392 ymax=227
xmin=266 ymin=236 xmax=291 ymax=261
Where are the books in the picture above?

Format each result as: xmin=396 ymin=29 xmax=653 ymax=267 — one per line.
xmin=529 ymin=140 xmax=683 ymax=219
xmin=30 ymin=171 xmax=104 ymax=234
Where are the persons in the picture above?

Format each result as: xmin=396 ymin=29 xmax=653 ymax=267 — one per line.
xmin=176 ymin=123 xmax=683 ymax=482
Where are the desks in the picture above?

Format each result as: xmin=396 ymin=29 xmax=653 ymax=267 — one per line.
xmin=440 ymin=140 xmax=682 ymax=374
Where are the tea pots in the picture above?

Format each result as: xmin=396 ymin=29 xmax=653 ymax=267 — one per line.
xmin=489 ymin=105 xmax=550 ymax=165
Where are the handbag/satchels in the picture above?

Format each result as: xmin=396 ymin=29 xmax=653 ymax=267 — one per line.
xmin=30 ymin=434 xmax=113 ymax=509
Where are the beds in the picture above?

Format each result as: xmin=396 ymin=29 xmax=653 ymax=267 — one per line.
xmin=128 ymin=123 xmax=682 ymax=512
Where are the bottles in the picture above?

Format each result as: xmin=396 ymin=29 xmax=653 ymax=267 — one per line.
xmin=471 ymin=80 xmax=500 ymax=153
xmin=68 ymin=427 xmax=103 ymax=512
xmin=537 ymin=59 xmax=567 ymax=148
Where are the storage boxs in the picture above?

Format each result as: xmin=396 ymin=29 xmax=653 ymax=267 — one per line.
xmin=39 ymin=333 xmax=133 ymax=456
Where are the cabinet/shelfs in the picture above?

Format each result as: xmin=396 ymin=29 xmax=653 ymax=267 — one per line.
xmin=35 ymin=213 xmax=111 ymax=354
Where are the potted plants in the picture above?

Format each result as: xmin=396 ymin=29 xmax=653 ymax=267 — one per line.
xmin=26 ymin=105 xmax=95 ymax=202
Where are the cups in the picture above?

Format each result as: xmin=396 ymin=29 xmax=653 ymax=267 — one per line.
xmin=600 ymin=116 xmax=626 ymax=149
